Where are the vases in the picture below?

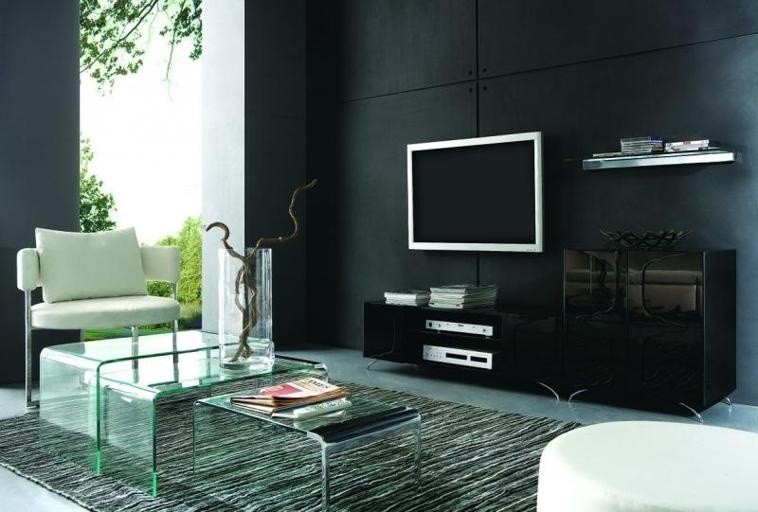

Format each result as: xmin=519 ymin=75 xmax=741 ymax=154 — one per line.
xmin=216 ymin=246 xmax=273 ymax=368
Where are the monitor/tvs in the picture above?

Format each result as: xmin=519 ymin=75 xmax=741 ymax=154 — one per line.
xmin=593 ymin=136 xmax=709 ymax=158
xmin=384 ymin=283 xmax=498 ymax=309
xmin=231 ymin=377 xmax=347 ymax=414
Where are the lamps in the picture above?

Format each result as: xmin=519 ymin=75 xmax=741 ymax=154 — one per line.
xmin=17 ymin=244 xmax=184 ymax=406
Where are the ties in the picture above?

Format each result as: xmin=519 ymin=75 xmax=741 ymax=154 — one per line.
xmin=407 ymin=131 xmax=543 ymax=253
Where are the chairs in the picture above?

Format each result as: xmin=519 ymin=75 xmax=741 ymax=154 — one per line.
xmin=17 ymin=244 xmax=184 ymax=406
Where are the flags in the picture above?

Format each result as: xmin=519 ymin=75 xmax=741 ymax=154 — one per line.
xmin=216 ymin=246 xmax=273 ymax=368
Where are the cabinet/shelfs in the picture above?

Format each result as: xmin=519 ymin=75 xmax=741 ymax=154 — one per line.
xmin=362 ymin=299 xmax=560 ymax=405
xmin=562 ymin=248 xmax=739 ymax=423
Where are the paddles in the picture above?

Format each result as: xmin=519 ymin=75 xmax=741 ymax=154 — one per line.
xmin=0 ymin=355 xmax=584 ymax=511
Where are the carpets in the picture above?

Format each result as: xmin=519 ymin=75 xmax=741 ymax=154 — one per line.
xmin=0 ymin=355 xmax=584 ymax=511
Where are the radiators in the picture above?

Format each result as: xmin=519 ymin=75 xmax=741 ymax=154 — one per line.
xmin=293 ymin=399 xmax=352 ymax=419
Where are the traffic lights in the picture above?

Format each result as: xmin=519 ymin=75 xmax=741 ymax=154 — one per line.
xmin=34 ymin=222 xmax=149 ymax=303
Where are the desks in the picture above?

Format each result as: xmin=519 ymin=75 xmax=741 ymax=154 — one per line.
xmin=38 ymin=330 xmax=426 ymax=512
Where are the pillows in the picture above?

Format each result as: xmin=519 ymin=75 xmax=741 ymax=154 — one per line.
xmin=34 ymin=222 xmax=149 ymax=303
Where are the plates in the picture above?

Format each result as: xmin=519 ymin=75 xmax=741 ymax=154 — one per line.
xmin=562 ymin=248 xmax=739 ymax=423
xmin=362 ymin=299 xmax=560 ymax=405
